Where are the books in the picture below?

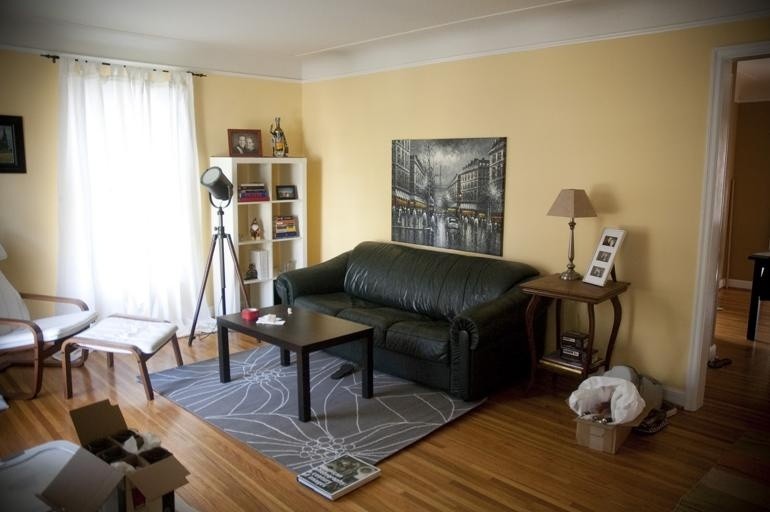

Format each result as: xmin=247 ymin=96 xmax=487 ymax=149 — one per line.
xmin=249 ymin=248 xmax=268 ymax=281
xmin=539 ymin=360 xmax=599 ymax=376
xmin=542 ymin=350 xmax=605 ymax=370
xmin=238 ymin=183 xmax=267 ymax=201
xmin=297 ymin=454 xmax=381 ymax=502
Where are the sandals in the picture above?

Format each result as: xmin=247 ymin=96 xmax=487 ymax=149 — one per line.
xmin=639 ymin=410 xmax=669 ymax=434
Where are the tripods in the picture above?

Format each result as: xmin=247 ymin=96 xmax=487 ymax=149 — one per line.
xmin=188 ymin=207 xmax=261 ymax=347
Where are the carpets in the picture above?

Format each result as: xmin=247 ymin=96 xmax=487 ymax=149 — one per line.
xmin=138 ymin=343 xmax=488 ymax=474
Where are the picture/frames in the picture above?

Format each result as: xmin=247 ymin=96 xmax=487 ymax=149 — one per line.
xmin=583 ymin=228 xmax=627 ymax=287
xmin=0 ymin=115 xmax=25 ymax=173
xmin=276 ymin=185 xmax=296 ymax=200
xmin=228 ymin=128 xmax=262 ymax=157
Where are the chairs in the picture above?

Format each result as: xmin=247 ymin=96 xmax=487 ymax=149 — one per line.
xmin=1 ymin=244 xmax=98 ymax=410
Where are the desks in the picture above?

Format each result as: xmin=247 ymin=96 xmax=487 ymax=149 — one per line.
xmin=747 ymin=252 xmax=770 ymax=341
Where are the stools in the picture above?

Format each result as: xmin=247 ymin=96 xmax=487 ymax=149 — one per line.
xmin=61 ymin=314 xmax=183 ymax=401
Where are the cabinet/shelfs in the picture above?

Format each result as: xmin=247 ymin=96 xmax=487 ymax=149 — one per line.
xmin=209 ymin=157 xmax=307 ymax=318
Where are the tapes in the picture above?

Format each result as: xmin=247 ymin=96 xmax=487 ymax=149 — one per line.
xmin=241 ymin=307 xmax=259 ymax=320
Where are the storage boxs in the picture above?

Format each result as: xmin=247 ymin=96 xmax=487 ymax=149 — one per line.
xmin=564 ymin=373 xmax=660 ymax=453
xmin=0 ymin=440 xmax=119 ymax=512
xmin=34 ymin=398 xmax=190 ymax=512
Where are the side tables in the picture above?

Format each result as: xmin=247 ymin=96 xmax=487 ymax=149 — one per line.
xmin=518 ymin=273 xmax=630 ymax=394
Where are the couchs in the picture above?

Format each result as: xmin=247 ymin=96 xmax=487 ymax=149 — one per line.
xmin=274 ymin=241 xmax=548 ymax=403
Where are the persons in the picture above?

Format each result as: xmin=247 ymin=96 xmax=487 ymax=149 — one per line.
xmin=244 ymin=137 xmax=256 ymax=153
xmin=233 ymin=134 xmax=245 ymax=154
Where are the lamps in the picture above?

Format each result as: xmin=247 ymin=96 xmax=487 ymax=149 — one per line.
xmin=547 ymin=189 xmax=598 ymax=282
